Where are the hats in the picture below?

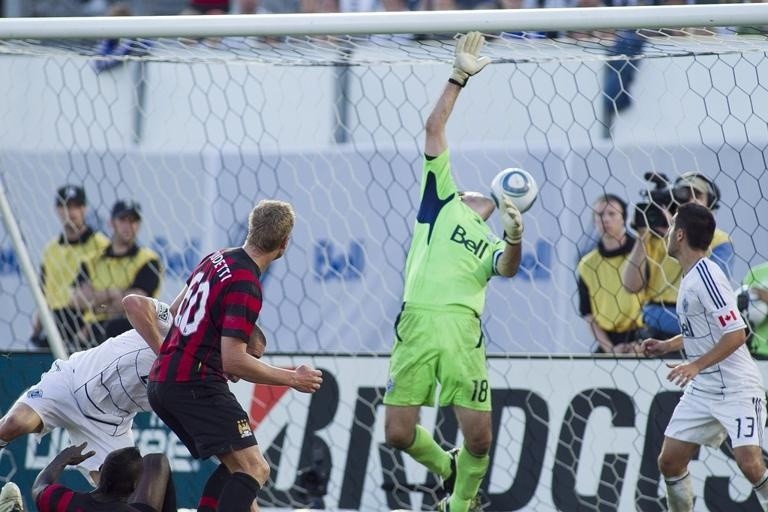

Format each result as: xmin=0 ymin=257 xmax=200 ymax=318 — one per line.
xmin=111 ymin=200 xmax=142 ymax=221
xmin=56 ymin=186 xmax=88 ymax=206
xmin=678 ymin=171 xmax=714 ymax=196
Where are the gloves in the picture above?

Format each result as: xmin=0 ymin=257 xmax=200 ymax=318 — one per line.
xmin=500 ymin=192 xmax=524 ymax=245
xmin=449 ymin=32 xmax=492 ymax=87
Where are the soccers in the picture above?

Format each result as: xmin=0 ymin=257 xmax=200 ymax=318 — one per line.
xmin=488 ymin=166 xmax=540 ymax=216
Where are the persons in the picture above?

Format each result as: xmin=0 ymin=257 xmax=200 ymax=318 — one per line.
xmin=29 ymin=184 xmax=113 ymax=348
xmin=621 ymin=171 xmax=736 ymax=358
xmin=577 ymin=195 xmax=647 ymax=354
xmin=383 ymin=31 xmax=523 ymax=512
xmin=68 ymin=200 xmax=162 ymax=348
xmin=641 ymin=203 xmax=768 ymax=512
xmin=0 ymin=441 xmax=177 ymax=512
xmin=0 ymin=295 xmax=268 ymax=488
xmin=737 ymin=260 xmax=768 ymax=361
xmin=147 ymin=200 xmax=324 ymax=512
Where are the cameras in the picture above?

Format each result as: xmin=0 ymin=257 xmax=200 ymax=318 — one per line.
xmin=635 ymin=174 xmax=691 ymax=228
